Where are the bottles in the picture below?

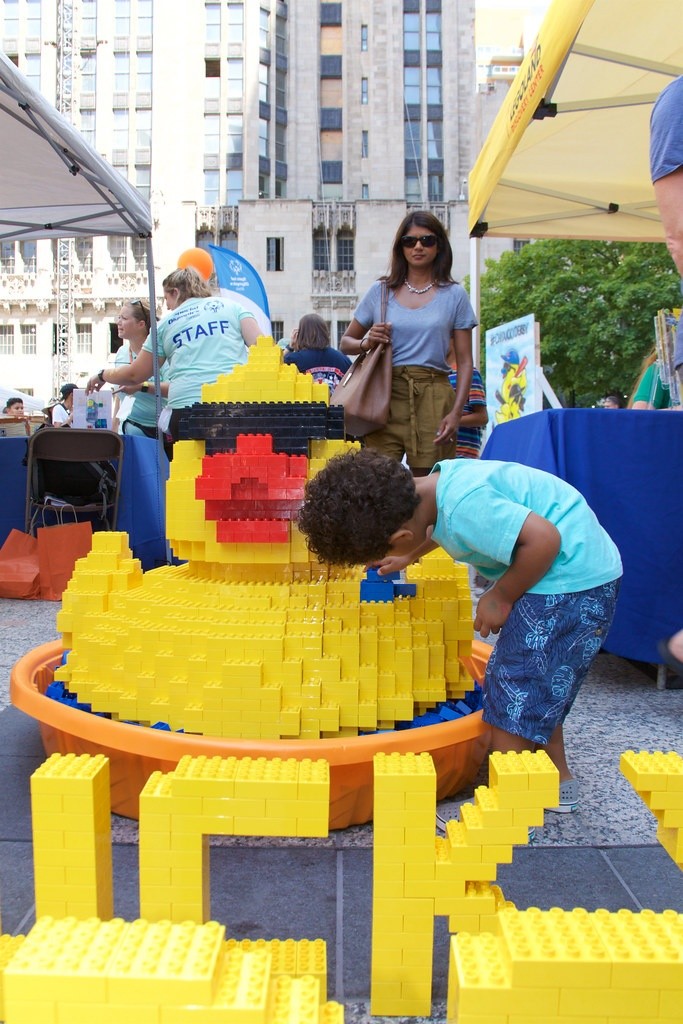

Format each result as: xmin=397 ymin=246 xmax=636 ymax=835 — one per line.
xmin=86 ymin=387 xmax=97 ymax=422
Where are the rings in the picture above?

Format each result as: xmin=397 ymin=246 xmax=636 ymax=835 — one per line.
xmin=450 ymin=438 xmax=454 ymax=442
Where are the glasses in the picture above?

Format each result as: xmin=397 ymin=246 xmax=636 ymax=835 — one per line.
xmin=130 ymin=299 xmax=148 ymax=322
xmin=401 ymin=235 xmax=437 ymax=248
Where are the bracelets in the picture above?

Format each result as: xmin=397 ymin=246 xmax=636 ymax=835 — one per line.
xmin=286 ymin=345 xmax=294 ymax=352
xmin=360 ymin=338 xmax=370 ymax=352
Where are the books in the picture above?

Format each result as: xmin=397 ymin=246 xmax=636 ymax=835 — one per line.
xmin=483 ymin=313 xmax=543 ymax=437
xmin=44 ymin=496 xmax=96 ymax=507
xmin=654 ymin=308 xmax=683 ymax=403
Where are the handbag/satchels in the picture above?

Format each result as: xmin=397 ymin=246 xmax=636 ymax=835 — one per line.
xmin=37 ymin=504 xmax=92 ymax=601
xmin=330 ymin=279 xmax=393 ymax=437
xmin=0 ymin=521 xmax=47 ymax=600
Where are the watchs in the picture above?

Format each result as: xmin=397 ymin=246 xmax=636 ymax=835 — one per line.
xmin=98 ymin=369 xmax=106 ymax=383
xmin=141 ymin=381 xmax=149 ymax=392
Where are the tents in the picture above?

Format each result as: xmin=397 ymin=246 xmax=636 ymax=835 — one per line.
xmin=465 ymin=1 xmax=683 ymax=409
xmin=0 ymin=44 xmax=173 ymax=569
xmin=0 ymin=386 xmax=45 ymax=416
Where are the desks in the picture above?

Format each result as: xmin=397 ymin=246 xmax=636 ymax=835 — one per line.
xmin=468 ymin=408 xmax=683 ymax=691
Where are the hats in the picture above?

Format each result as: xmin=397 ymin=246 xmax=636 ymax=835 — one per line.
xmin=59 ymin=384 xmax=79 ymax=401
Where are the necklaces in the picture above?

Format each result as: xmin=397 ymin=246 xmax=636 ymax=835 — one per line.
xmin=403 ymin=277 xmax=435 ymax=294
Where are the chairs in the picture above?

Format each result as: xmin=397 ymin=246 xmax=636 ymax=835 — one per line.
xmin=26 ymin=428 xmax=125 ymax=534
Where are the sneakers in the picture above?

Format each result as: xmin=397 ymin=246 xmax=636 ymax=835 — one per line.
xmin=436 ymin=797 xmax=535 ymax=843
xmin=544 ymin=779 xmax=580 ymax=814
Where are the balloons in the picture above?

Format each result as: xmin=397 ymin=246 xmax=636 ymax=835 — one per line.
xmin=178 ymin=248 xmax=213 ymax=281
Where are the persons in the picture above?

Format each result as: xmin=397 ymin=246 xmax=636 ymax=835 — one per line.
xmin=338 ymin=211 xmax=474 ymax=475
xmin=623 ymin=344 xmax=674 ymax=412
xmin=299 ymin=448 xmax=624 ymax=836
xmin=283 ymin=313 xmax=353 ymax=399
xmin=646 ymin=75 xmax=683 ymax=677
xmin=604 ymin=396 xmax=620 ymax=409
xmin=52 ymin=384 xmax=79 ymax=430
xmin=33 ymin=396 xmax=72 ymax=432
xmin=443 ymin=324 xmax=490 ymax=459
xmin=7 ymin=398 xmax=25 ymax=416
xmin=82 ymin=264 xmax=264 ymax=567
